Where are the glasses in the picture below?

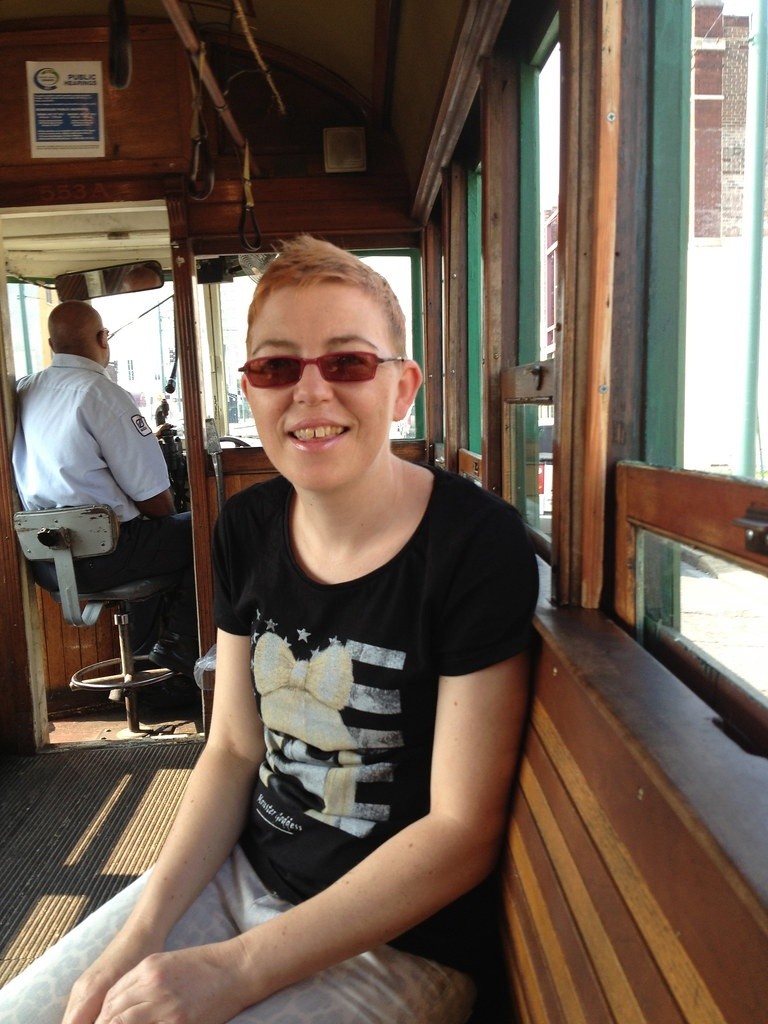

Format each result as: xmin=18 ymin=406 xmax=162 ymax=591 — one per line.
xmin=238 ymin=351 xmax=404 ymax=388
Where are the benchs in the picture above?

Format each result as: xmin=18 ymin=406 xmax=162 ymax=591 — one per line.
xmin=492 ymin=555 xmax=768 ymax=1024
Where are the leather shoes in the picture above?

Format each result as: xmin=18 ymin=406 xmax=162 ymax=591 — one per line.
xmin=148 ymin=632 xmax=198 ymax=678
xmin=139 ymin=683 xmax=201 ymax=707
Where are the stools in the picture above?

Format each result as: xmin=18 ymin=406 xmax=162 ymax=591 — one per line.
xmin=12 ymin=502 xmax=175 ymax=740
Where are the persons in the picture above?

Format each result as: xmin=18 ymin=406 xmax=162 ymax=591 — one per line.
xmin=123 ymin=267 xmax=161 ymax=291
xmin=0 ymin=234 xmax=541 ymax=1024
xmin=10 ymin=299 xmax=200 ymax=674
